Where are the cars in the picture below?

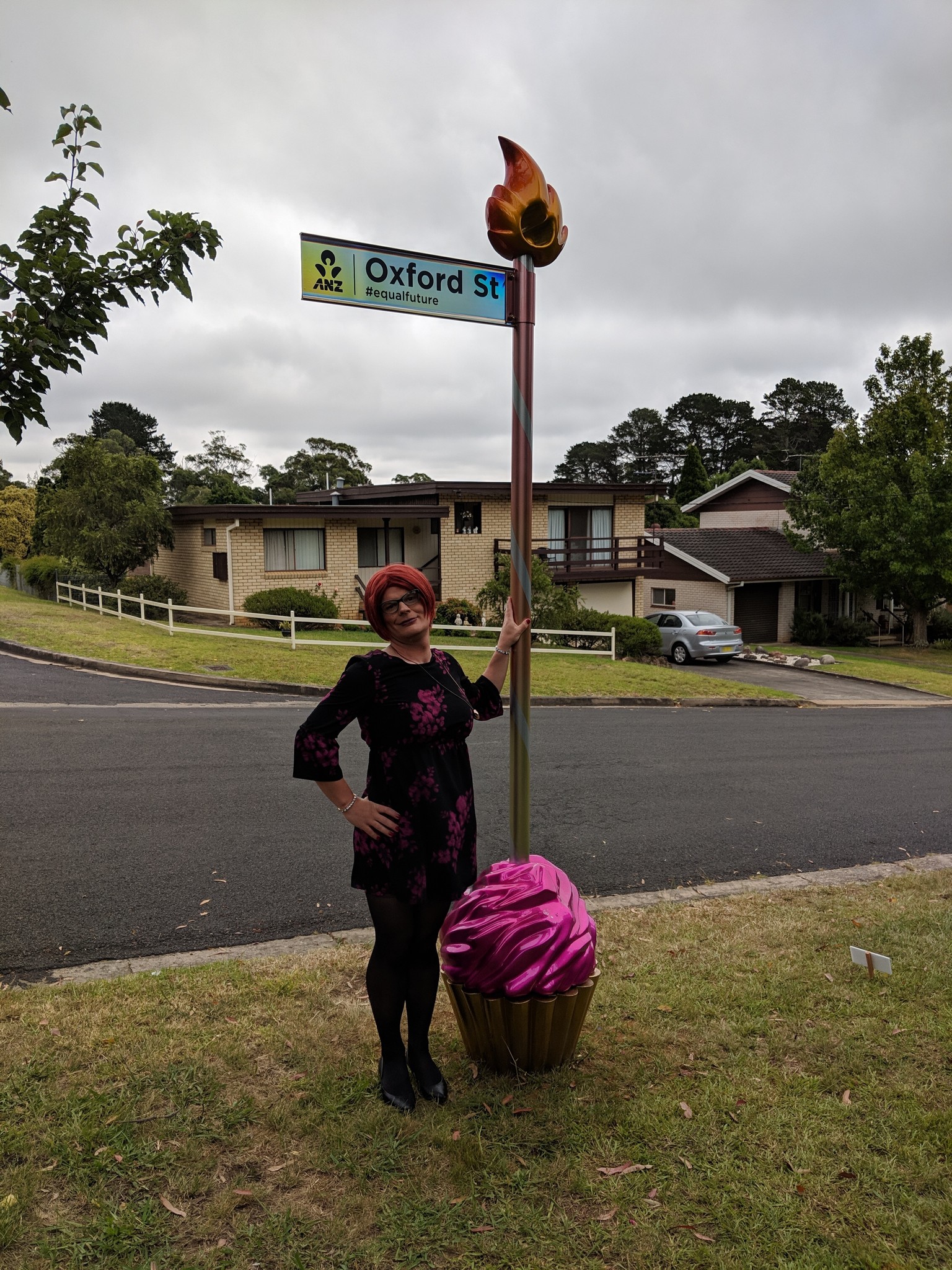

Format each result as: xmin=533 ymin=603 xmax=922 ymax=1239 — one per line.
xmin=637 ymin=608 xmax=744 ymax=665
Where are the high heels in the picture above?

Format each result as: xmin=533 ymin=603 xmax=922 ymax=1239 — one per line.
xmin=379 ymin=1056 xmax=415 ymax=1112
xmin=405 ymin=1048 xmax=448 ymax=1103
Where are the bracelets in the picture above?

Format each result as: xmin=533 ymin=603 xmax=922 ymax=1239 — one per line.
xmin=495 ymin=646 xmax=511 ymax=654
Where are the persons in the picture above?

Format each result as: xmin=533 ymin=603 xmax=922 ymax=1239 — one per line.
xmin=292 ymin=565 xmax=532 ymax=1112
xmin=463 ymin=617 xmax=472 ymax=626
xmin=454 ymin=614 xmax=462 ymax=625
xmin=481 ymin=613 xmax=486 ymax=627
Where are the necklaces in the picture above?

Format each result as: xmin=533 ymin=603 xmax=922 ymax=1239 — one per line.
xmin=338 ymin=793 xmax=357 ymax=813
xmin=391 ymin=646 xmax=480 ymax=719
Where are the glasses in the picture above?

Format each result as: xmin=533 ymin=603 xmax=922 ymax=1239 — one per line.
xmin=379 ymin=588 xmax=419 ymax=615
